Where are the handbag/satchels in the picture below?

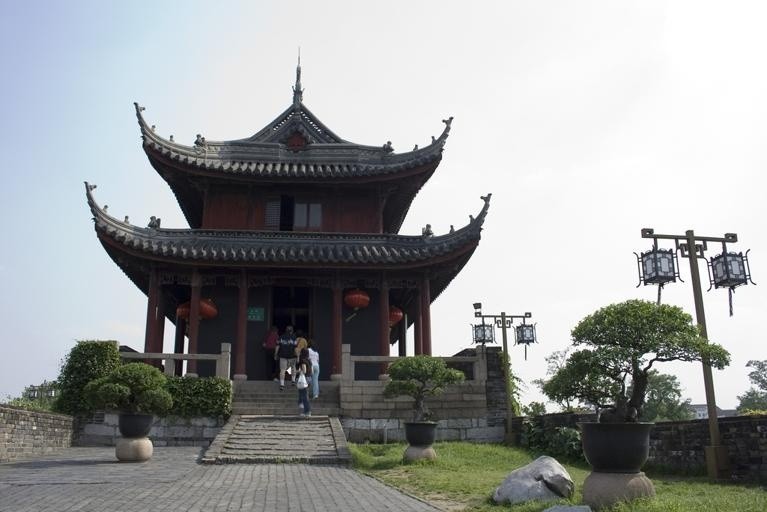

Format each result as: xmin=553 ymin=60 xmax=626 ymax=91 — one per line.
xmin=297 ymin=372 xmax=309 ymax=391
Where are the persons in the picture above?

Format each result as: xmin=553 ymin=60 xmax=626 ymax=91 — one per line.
xmin=262 ymin=326 xmax=320 ymax=417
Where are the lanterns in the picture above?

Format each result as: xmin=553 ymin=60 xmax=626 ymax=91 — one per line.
xmin=512 ymin=322 xmax=539 ymax=346
xmin=177 ymin=303 xmax=190 ymax=319
xmin=470 ymin=323 xmax=497 ymax=345
xmin=199 ymin=298 xmax=218 ymax=319
xmin=389 ymin=306 xmax=403 ymax=326
xmin=633 ymin=245 xmax=684 ymax=289
xmin=344 ymin=289 xmax=370 ymax=310
xmin=704 ymin=249 xmax=757 ymax=293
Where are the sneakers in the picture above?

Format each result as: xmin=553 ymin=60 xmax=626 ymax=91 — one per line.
xmin=280 ymin=381 xmax=297 ymax=391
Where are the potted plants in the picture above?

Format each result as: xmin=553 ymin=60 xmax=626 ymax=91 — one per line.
xmin=383 ymin=353 xmax=465 ymax=446
xmin=84 ymin=362 xmax=174 ymax=437
xmin=540 ymin=296 xmax=732 ymax=474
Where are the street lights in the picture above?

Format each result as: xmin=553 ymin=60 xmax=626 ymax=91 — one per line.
xmin=29 ymin=384 xmax=57 ymax=407
xmin=470 ymin=303 xmax=539 ymax=442
xmin=633 ymin=228 xmax=756 ymax=479
